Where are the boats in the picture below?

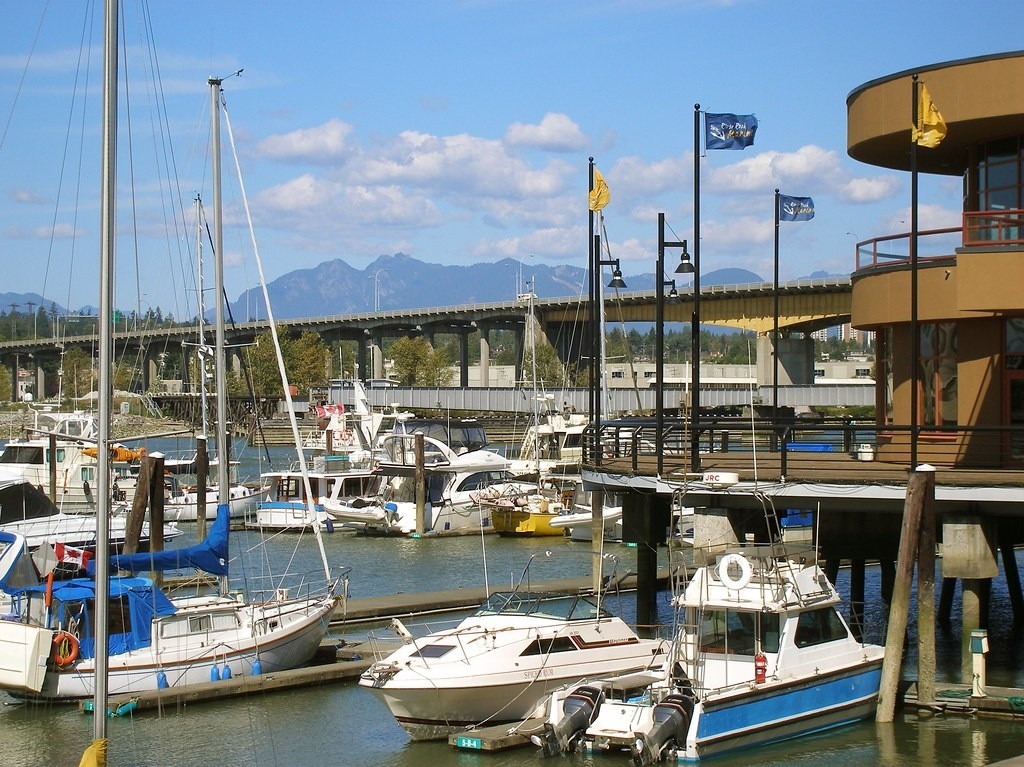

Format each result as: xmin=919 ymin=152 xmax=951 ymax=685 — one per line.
xmin=528 ymin=492 xmax=907 ymax=765
xmin=355 ymin=549 xmax=679 ymax=743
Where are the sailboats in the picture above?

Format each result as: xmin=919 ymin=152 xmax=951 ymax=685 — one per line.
xmin=1 ymin=1 xmax=697 ymax=703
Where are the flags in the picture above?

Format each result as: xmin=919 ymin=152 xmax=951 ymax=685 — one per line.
xmin=315 ymin=403 xmax=344 ymax=418
xmin=778 ymin=193 xmax=815 ymax=222
xmin=54 ymin=542 xmax=92 ymax=570
xmin=588 ymin=165 xmax=611 ymax=213
xmin=702 ymin=110 xmax=759 ymax=151
xmin=910 ymin=84 xmax=948 ymax=148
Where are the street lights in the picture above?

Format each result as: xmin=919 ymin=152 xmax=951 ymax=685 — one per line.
xmin=375 ymin=269 xmax=389 ymax=313
xmin=246 ymin=281 xmax=261 ymax=321
xmin=519 ymin=252 xmax=535 ymax=298
xmin=593 ymin=235 xmax=628 ymax=463
xmin=655 ymin=211 xmax=696 ymax=476
xmin=367 ymin=275 xmax=382 ymax=311
xmin=506 ymin=263 xmax=518 ymax=300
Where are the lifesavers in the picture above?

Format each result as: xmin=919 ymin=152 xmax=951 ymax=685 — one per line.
xmin=719 ymin=554 xmax=751 ymax=591
xmin=139 ymin=448 xmax=148 ymax=460
xmin=340 ymin=430 xmax=350 ymax=441
xmin=51 ymin=633 xmax=79 ymax=664
